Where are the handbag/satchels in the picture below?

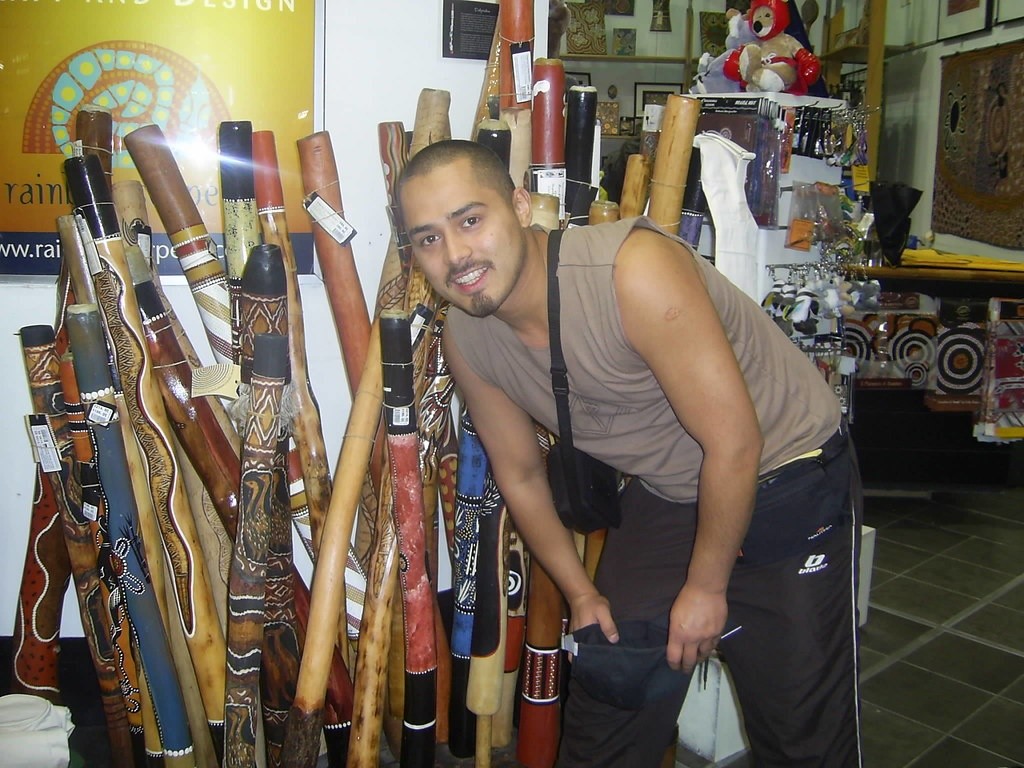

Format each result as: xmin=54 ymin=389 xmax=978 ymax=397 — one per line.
xmin=547 ymin=441 xmax=624 ymax=533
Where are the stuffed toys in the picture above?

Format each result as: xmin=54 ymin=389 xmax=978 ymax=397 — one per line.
xmin=688 ymin=0 xmax=822 ymax=95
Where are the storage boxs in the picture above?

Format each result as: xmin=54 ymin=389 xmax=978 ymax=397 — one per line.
xmin=596 ymin=102 xmax=620 ymax=135
xmin=642 ymin=104 xmax=667 ymax=132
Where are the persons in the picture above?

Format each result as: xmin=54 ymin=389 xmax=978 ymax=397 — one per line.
xmin=391 ymin=139 xmax=865 ymax=768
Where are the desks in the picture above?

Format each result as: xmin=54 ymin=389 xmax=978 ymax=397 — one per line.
xmin=856 ymin=249 xmax=1023 ymax=283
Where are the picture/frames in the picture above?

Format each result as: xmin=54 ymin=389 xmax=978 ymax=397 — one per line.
xmin=634 ymin=83 xmax=685 ymax=119
xmin=997 ymin=0 xmax=1024 ymax=23
xmin=565 ymin=71 xmax=592 ymax=87
xmin=936 ymin=0 xmax=994 ymax=41
